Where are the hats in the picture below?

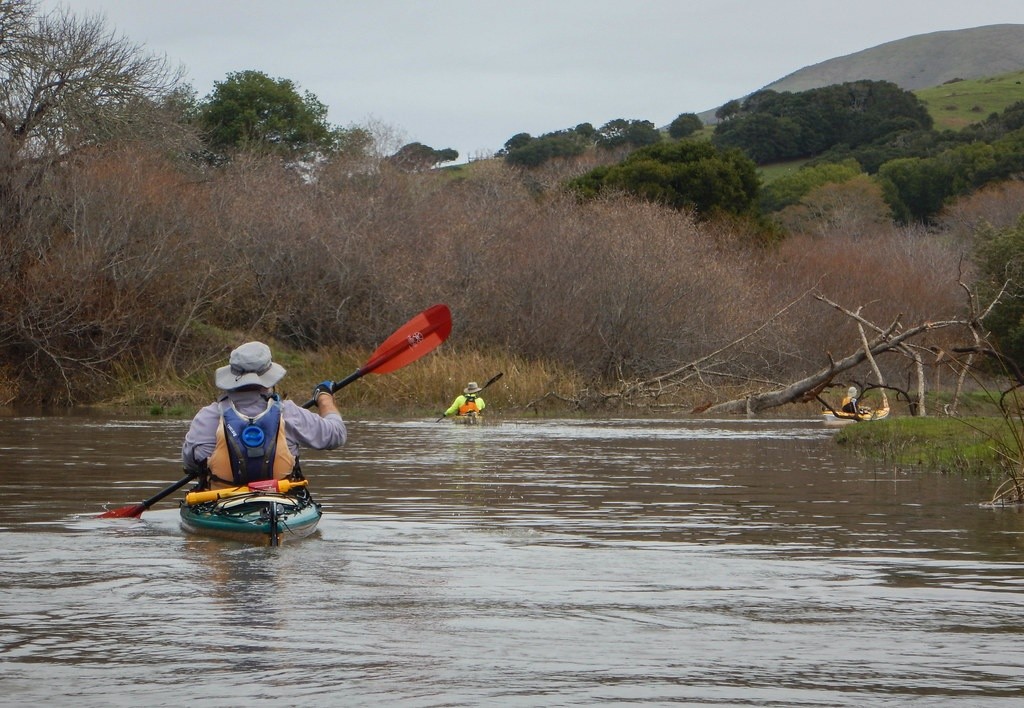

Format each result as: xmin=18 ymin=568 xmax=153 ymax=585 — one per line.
xmin=464 ymin=382 xmax=482 ymax=393
xmin=215 ymin=341 xmax=287 ymax=390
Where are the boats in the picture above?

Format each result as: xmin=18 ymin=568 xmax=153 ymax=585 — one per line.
xmin=822 ymin=408 xmax=889 ymax=423
xmin=452 ymin=409 xmax=483 ymax=427
xmin=179 ymin=482 xmax=322 ymax=548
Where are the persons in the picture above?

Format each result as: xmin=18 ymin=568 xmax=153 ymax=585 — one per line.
xmin=443 ymin=382 xmax=485 ymax=417
xmin=182 ymin=341 xmax=348 ymax=501
xmin=842 ymin=387 xmax=871 ymax=417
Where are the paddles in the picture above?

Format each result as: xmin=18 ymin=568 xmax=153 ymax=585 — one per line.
xmin=105 ymin=302 xmax=454 ymax=519
xmin=436 ymin=373 xmax=504 ymax=422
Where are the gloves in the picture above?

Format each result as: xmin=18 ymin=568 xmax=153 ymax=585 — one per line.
xmin=313 ymin=380 xmax=336 ymax=407
